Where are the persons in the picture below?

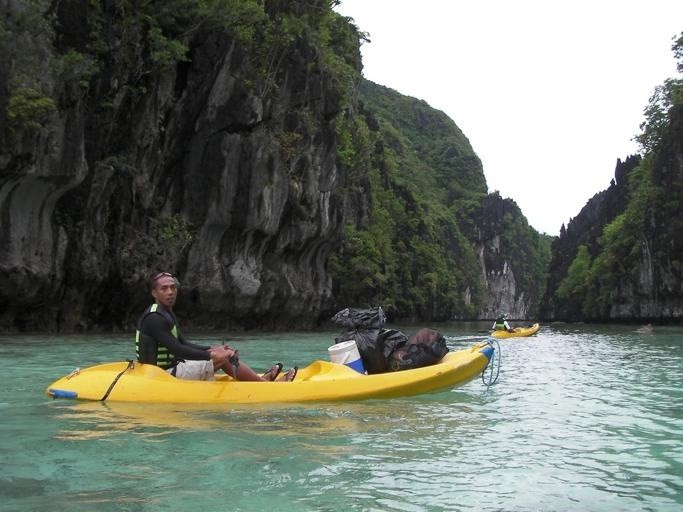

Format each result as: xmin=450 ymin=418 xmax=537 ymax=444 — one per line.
xmin=135 ymin=271 xmax=298 ymax=383
xmin=491 ymin=314 xmax=510 ymax=331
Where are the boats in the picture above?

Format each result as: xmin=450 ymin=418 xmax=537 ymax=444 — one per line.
xmin=46 ymin=338 xmax=495 ymax=414
xmin=487 ymin=323 xmax=539 ymax=340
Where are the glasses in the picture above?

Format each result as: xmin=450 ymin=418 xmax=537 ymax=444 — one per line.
xmin=154 ymin=272 xmax=172 ymax=280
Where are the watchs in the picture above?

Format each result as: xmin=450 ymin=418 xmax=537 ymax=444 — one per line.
xmin=210 ymin=351 xmax=216 ymax=359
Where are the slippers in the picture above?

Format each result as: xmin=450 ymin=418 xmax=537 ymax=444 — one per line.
xmin=262 ymin=363 xmax=298 ymax=382
